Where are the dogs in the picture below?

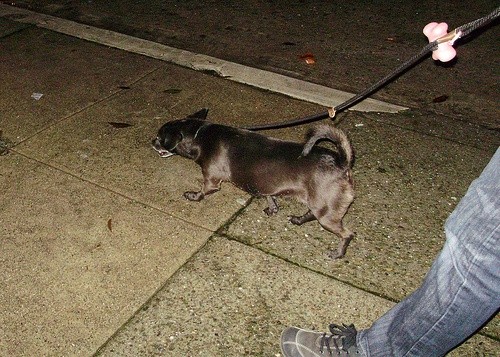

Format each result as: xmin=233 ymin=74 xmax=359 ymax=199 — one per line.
xmin=151 ymin=107 xmax=356 ymax=260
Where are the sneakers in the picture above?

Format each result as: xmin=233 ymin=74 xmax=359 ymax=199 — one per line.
xmin=279 ymin=323 xmax=359 ymax=357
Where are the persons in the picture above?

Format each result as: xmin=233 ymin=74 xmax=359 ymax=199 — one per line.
xmin=280 ymin=144 xmax=500 ymax=357
